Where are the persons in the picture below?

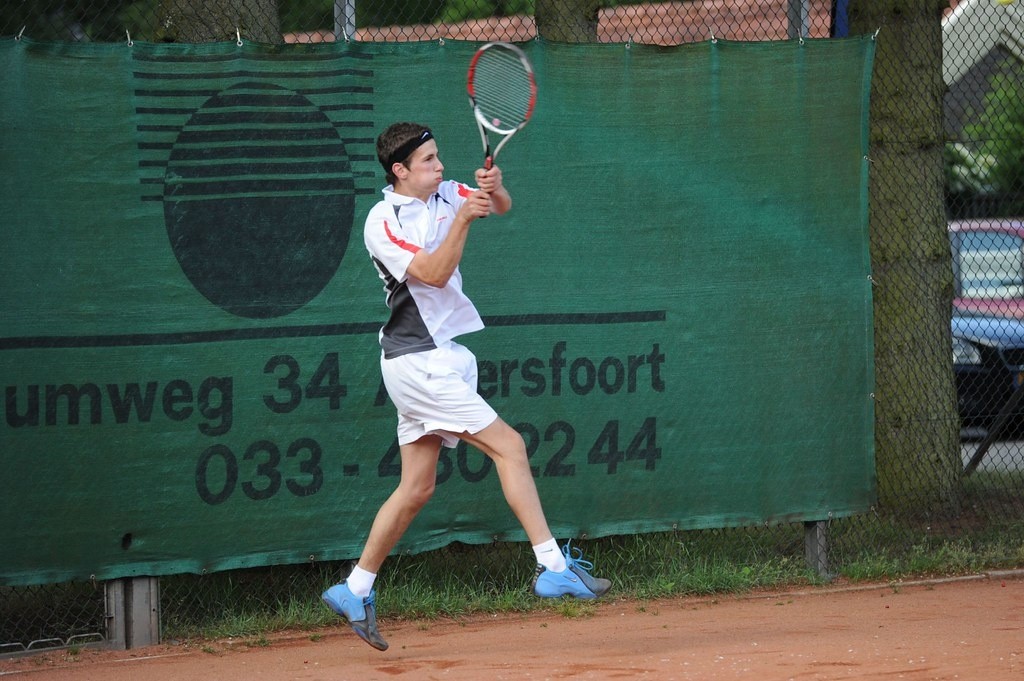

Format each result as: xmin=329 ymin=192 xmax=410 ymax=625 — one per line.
xmin=318 ymin=122 xmax=611 ymax=653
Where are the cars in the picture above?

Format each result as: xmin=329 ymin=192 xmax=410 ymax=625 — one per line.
xmin=948 ymin=220 xmax=1024 ymax=440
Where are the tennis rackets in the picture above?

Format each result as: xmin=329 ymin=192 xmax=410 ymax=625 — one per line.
xmin=466 ymin=41 xmax=537 ymax=218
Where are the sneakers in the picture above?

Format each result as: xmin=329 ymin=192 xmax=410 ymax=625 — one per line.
xmin=321 ymin=580 xmax=390 ymax=652
xmin=531 ymin=539 xmax=609 ymax=600
